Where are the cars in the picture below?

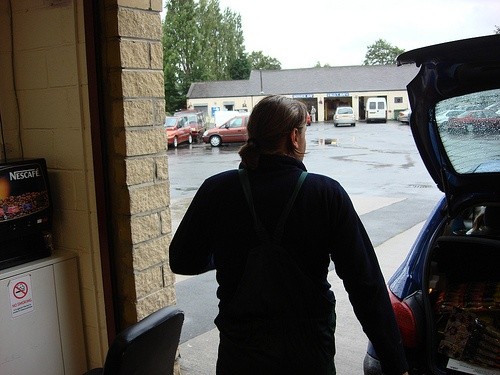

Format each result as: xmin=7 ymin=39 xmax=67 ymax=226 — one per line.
xmin=334 ymin=106 xmax=356 ymax=129
xmin=172 ymin=110 xmax=207 ymax=143
xmin=199 ymin=113 xmax=249 ymax=147
xmin=165 ymin=114 xmax=194 ymax=149
xmin=398 ymin=107 xmax=500 ymax=134
xmin=361 ymin=32 xmax=499 ymax=372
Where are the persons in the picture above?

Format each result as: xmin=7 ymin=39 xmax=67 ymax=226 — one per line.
xmin=309 ymin=106 xmax=316 ymax=122
xmin=168 ymin=94 xmax=411 ymax=375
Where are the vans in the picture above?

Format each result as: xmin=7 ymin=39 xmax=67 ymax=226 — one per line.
xmin=363 ymin=96 xmax=389 ymax=123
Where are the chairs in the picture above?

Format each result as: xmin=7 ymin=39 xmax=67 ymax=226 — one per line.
xmin=81 ymin=306 xmax=186 ymax=375
